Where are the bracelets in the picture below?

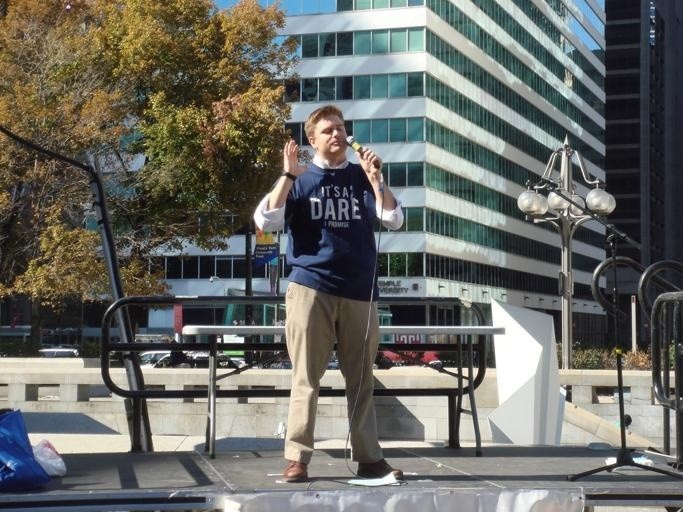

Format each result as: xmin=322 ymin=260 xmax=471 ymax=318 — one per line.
xmin=374 ymin=184 xmax=387 ymax=195
xmin=281 ymin=171 xmax=298 ymax=182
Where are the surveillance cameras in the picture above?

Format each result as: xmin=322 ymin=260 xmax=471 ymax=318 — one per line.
xmin=210 ymin=277 xmax=214 ymax=283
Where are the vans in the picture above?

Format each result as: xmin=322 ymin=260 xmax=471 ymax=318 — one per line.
xmin=38 ymin=348 xmax=79 ymax=357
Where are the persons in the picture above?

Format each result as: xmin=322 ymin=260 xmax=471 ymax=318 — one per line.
xmin=253 ymin=104 xmax=404 ymax=486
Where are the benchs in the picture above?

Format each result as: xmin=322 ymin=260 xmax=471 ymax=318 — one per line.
xmin=179 ymin=324 xmax=506 ymax=459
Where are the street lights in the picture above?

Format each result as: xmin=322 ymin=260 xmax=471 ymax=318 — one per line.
xmin=518 ymin=135 xmax=618 ymax=401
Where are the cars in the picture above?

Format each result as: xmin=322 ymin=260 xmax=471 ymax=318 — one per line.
xmin=114 ymin=339 xmax=379 ymax=369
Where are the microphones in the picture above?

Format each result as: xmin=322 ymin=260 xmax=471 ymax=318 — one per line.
xmin=345 ymin=135 xmax=382 ymax=170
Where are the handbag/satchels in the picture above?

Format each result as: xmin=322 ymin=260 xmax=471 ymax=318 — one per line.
xmin=0 ymin=407 xmax=52 ymax=491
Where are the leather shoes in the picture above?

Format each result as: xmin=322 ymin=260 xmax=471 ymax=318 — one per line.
xmin=357 ymin=459 xmax=404 ymax=481
xmin=284 ymin=462 xmax=309 ymax=483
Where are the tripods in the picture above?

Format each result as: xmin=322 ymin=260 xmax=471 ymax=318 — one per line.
xmin=529 ymin=172 xmax=683 ymax=482
